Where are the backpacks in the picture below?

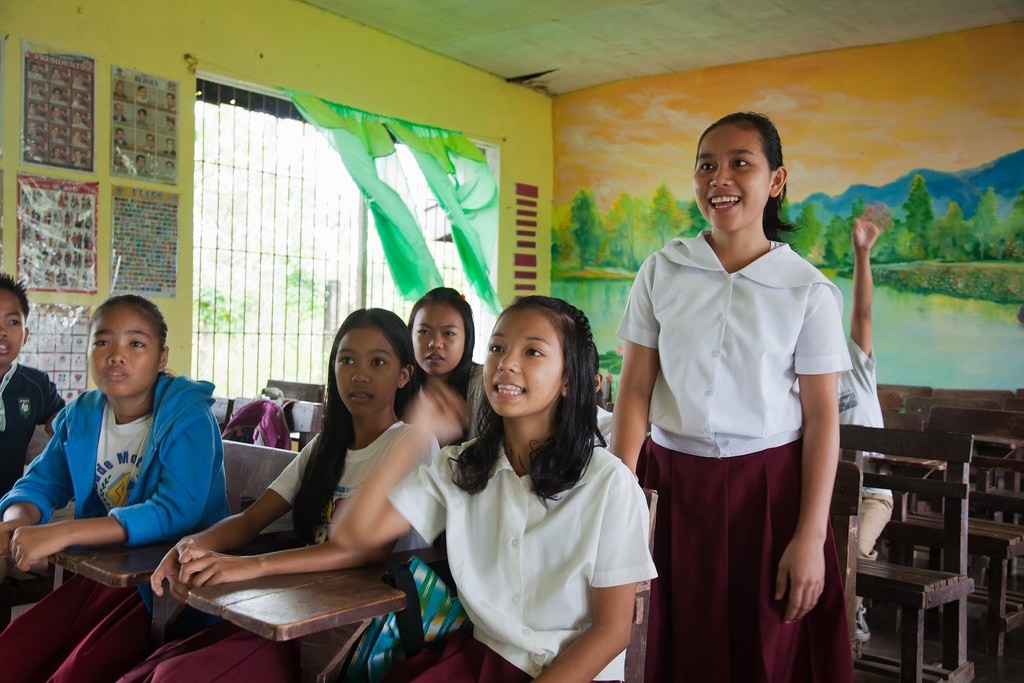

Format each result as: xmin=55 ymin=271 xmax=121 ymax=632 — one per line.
xmin=219 ymin=397 xmax=292 ymax=451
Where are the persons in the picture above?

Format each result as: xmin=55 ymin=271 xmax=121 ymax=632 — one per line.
xmin=116 ymin=287 xmax=658 ymax=683
xmin=609 ymin=112 xmax=854 ymax=683
xmin=838 ymin=203 xmax=895 ymax=641
xmin=0 ymin=272 xmax=231 ymax=683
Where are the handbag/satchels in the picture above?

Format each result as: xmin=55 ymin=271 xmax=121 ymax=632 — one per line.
xmin=343 ymin=555 xmax=472 ymax=683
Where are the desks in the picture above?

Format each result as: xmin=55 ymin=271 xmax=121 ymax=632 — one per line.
xmin=169 ymin=531 xmax=408 ymax=683
xmin=11 ymin=519 xmax=201 ymax=656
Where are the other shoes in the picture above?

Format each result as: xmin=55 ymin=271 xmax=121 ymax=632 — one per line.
xmin=854 ymin=609 xmax=870 ymax=642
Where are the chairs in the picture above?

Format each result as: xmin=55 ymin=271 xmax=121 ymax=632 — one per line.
xmin=231 ymin=397 xmax=324 ymax=452
xmin=268 ymin=379 xmax=325 ymax=404
xmin=211 ymin=398 xmax=232 ymax=439
xmin=829 ymin=384 xmax=1024 ymax=683
xmin=220 ymin=439 xmax=300 ymax=543
xmin=363 ymin=454 xmax=658 ymax=683
